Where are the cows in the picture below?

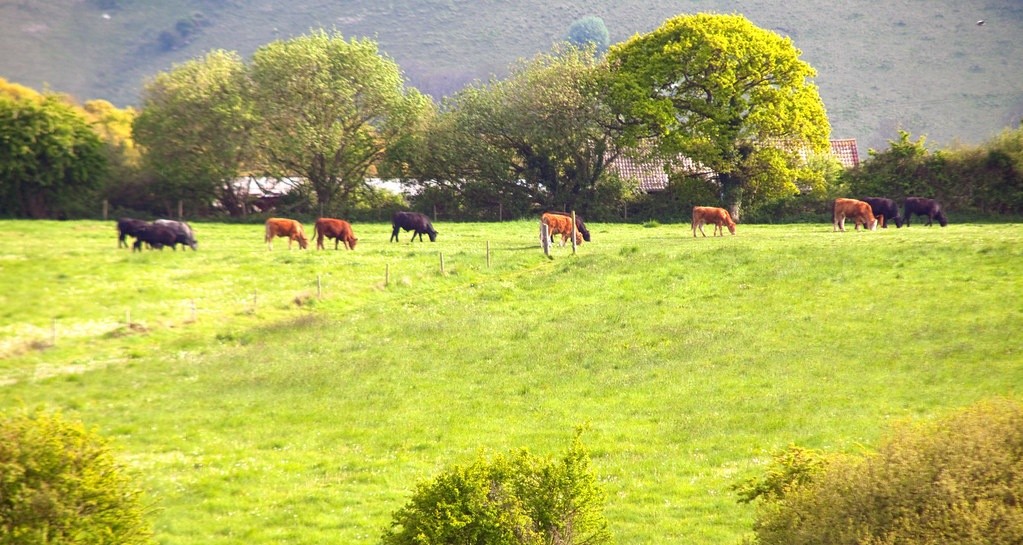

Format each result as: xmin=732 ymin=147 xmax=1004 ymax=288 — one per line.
xmin=541 ymin=210 xmax=591 ymax=245
xmin=311 ymin=218 xmax=358 ymax=250
xmin=390 ymin=211 xmax=439 ymax=243
xmin=690 ymin=205 xmax=737 ymax=238
xmin=831 ymin=196 xmax=948 ymax=232
xmin=265 ymin=217 xmax=308 ymax=250
xmin=117 ymin=217 xmax=198 ymax=251
